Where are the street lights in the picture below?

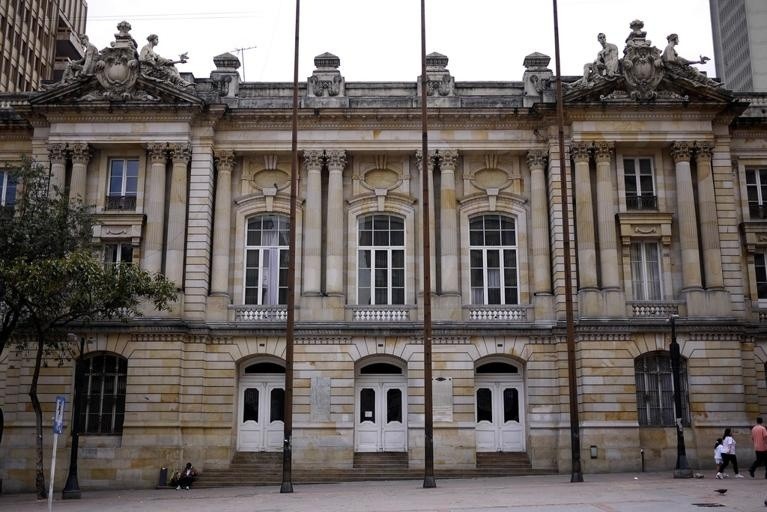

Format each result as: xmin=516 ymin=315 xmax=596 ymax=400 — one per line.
xmin=62 ymin=332 xmax=97 ymax=499
xmin=665 ymin=314 xmax=692 ymax=478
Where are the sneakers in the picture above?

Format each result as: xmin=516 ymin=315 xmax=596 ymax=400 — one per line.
xmin=735 ymin=473 xmax=745 ymax=478
xmin=748 ymin=469 xmax=755 ymax=478
xmin=186 ymin=486 xmax=189 ymax=490
xmin=715 ymin=472 xmax=729 ymax=479
xmin=176 ymin=485 xmax=181 ymax=489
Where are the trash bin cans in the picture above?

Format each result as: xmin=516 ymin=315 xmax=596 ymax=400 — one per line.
xmin=159 ymin=467 xmax=167 ymax=486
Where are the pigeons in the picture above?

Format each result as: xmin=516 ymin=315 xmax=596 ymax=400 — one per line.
xmin=179 ymin=52 xmax=189 ymax=61
xmin=700 ymin=55 xmax=711 ymax=62
xmin=600 ymin=54 xmax=604 ymax=64
xmin=714 ymin=489 xmax=727 ymax=495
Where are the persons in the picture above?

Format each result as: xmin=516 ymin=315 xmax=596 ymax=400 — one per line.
xmin=663 ymin=34 xmax=725 ymax=87
xmin=749 ymin=417 xmax=767 ymax=479
xmin=58 ymin=34 xmax=98 ymax=84
xmin=139 ymin=34 xmax=198 ymax=88
xmin=714 ymin=428 xmax=744 ymax=479
xmin=176 ymin=462 xmax=198 ymax=490
xmin=582 ymin=33 xmax=618 ymax=83
